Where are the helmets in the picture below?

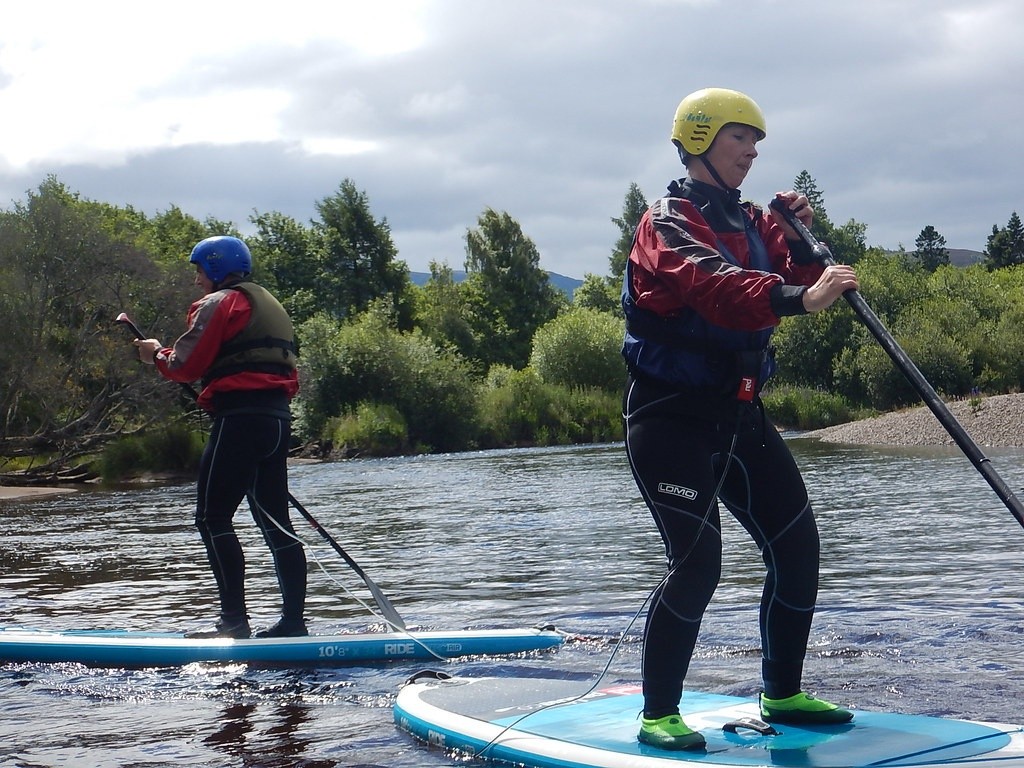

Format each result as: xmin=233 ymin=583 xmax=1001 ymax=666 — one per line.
xmin=672 ymin=87 xmax=766 ymax=156
xmin=191 ymin=236 xmax=251 ymax=284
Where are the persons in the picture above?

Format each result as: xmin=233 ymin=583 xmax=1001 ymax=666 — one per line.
xmin=135 ymin=236 xmax=308 ymax=640
xmin=621 ymin=87 xmax=859 ymax=749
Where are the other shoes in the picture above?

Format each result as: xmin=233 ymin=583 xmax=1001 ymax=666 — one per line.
xmin=257 ymin=618 xmax=306 ymax=637
xmin=637 ymin=714 xmax=706 ymax=750
xmin=184 ymin=615 xmax=250 ymax=639
xmin=759 ymin=691 xmax=853 ymax=724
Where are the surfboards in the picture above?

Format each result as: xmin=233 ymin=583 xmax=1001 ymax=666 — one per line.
xmin=392 ymin=663 xmax=1023 ymax=768
xmin=1 ymin=624 xmax=566 ymax=664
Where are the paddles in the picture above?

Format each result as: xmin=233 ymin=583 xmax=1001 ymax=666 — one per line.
xmin=116 ymin=312 xmax=406 ymax=633
xmin=769 ymin=193 xmax=1023 ymax=529
